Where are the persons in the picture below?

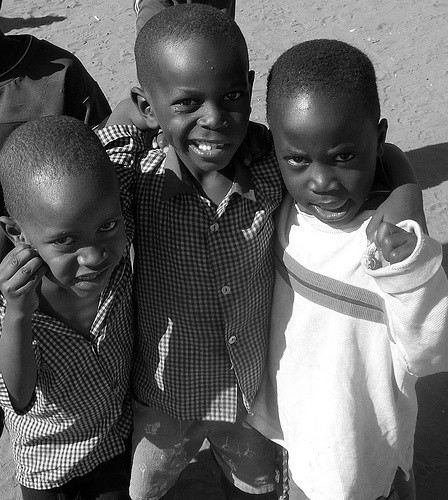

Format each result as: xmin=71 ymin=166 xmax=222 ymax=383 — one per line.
xmin=122 ymin=3 xmax=429 ymax=500
xmin=0 ymin=0 xmax=112 ymax=259
xmin=265 ymin=39 xmax=448 ymax=500
xmin=132 ymin=0 xmax=236 ymax=36
xmin=0 ymin=96 xmax=150 ymax=500
